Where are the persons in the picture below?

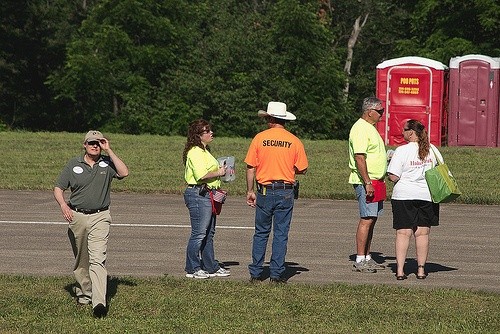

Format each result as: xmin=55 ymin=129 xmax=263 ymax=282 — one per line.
xmin=54 ymin=131 xmax=130 ymax=318
xmin=386 ymin=120 xmax=446 ymax=280
xmin=245 ymin=101 xmax=308 ymax=287
xmin=182 ymin=121 xmax=230 ymax=279
xmin=350 ymin=97 xmax=392 ymax=274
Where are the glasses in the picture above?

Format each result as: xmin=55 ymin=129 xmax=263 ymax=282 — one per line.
xmin=367 ymin=109 xmax=383 ymax=115
xmin=199 ymin=126 xmax=210 ymax=134
xmin=87 ymin=142 xmax=100 ymax=146
xmin=404 ymin=128 xmax=411 ymax=131
xmin=69 ymin=204 xmax=109 ymax=214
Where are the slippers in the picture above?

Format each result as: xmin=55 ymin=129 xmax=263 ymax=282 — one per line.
xmin=417 ymin=274 xmax=426 ymax=279
xmin=397 ymin=274 xmax=408 ymax=280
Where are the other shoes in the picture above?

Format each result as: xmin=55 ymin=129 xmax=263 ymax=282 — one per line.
xmin=92 ymin=302 xmax=107 ymax=317
xmin=270 ymin=276 xmax=280 ymax=283
xmin=250 ymin=276 xmax=262 ymax=282
xmin=77 ymin=295 xmax=91 ymax=305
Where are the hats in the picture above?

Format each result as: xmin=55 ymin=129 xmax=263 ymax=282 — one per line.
xmin=82 ymin=130 xmax=104 ymax=144
xmin=257 ymin=101 xmax=297 ymax=121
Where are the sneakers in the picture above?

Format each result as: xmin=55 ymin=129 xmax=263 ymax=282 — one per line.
xmin=352 ymin=258 xmax=386 ymax=273
xmin=209 ymin=267 xmax=231 ymax=277
xmin=185 ymin=269 xmax=209 ymax=279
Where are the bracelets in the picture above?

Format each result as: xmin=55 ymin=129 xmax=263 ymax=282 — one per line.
xmin=364 ymin=181 xmax=373 ymax=185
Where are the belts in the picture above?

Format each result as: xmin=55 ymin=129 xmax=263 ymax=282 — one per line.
xmin=189 ymin=184 xmax=202 ymax=188
xmin=266 ymin=185 xmax=292 ymax=189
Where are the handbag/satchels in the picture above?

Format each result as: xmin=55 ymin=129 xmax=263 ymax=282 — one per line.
xmin=370 ymin=179 xmax=386 ymax=202
xmin=208 ymin=189 xmax=227 ymax=215
xmin=424 ymin=144 xmax=462 ymax=204
